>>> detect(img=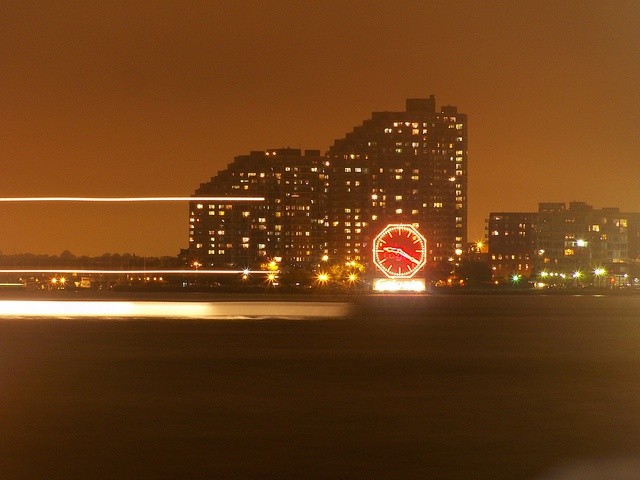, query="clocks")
[373,224,427,280]
